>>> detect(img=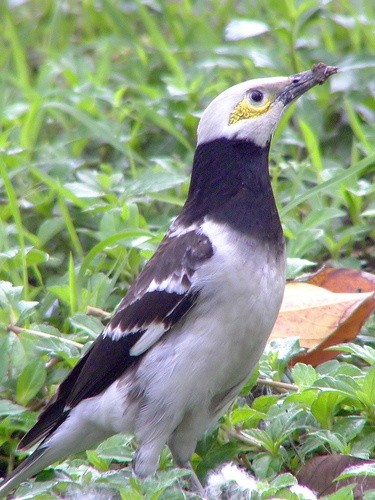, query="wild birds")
[1,61,339,500]
